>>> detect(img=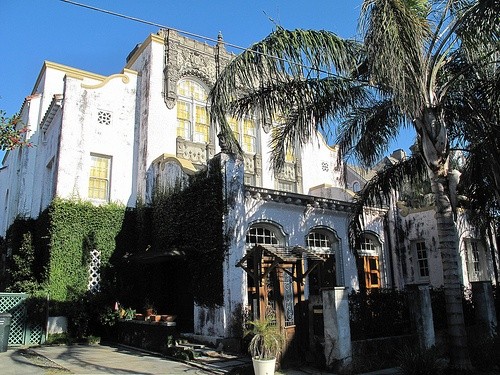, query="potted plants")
[242,315,287,375]
